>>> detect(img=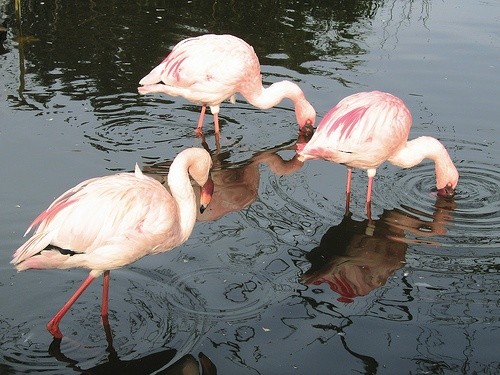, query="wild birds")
[9,147,214,339]
[137,34,316,138]
[128,133,307,222]
[295,90,459,202]
[297,203,458,305]
